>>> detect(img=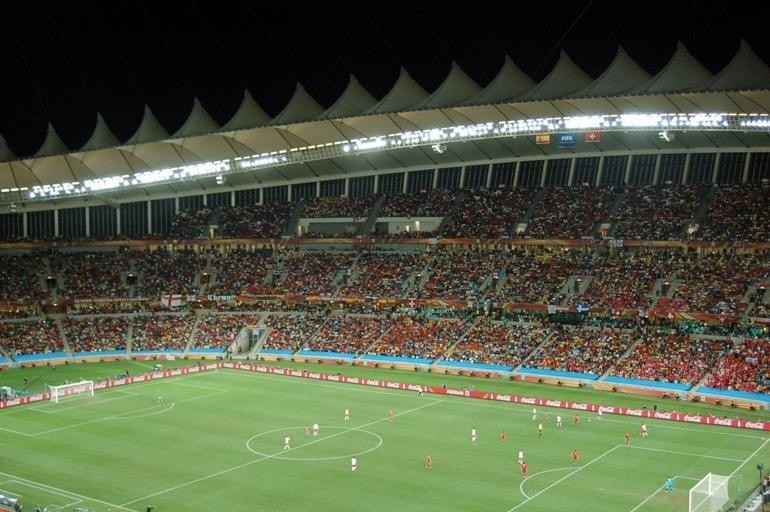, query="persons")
[313,421,319,436]
[521,461,527,479]
[570,449,579,465]
[283,434,291,450]
[574,413,579,424]
[499,430,506,443]
[640,421,644,435]
[624,431,631,448]
[665,475,675,494]
[35,503,41,511]
[537,421,544,435]
[544,409,550,423]
[518,447,524,465]
[641,421,649,439]
[146,503,157,511]
[426,453,433,469]
[597,404,604,421]
[556,413,562,428]
[157,395,163,407]
[418,387,424,400]
[0,181,769,395]
[344,406,349,422]
[14,498,24,512]
[387,406,393,418]
[471,426,476,442]
[305,422,310,436]
[532,406,538,421]
[351,455,358,471]
